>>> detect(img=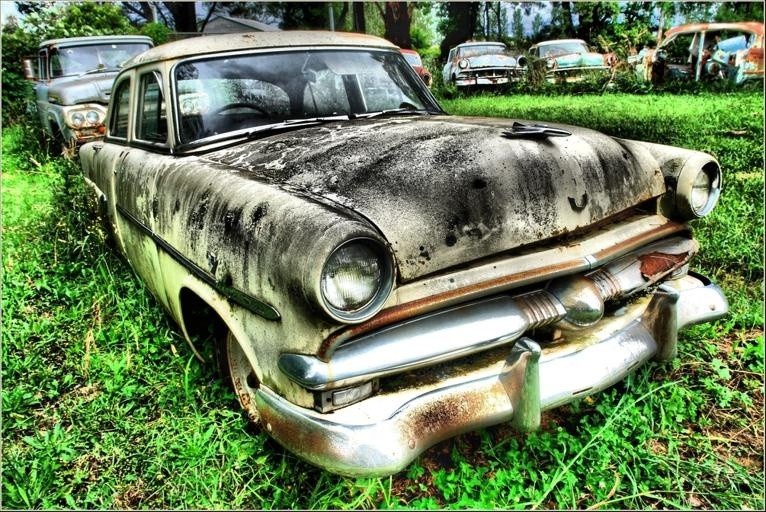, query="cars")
[71,29,733,479]
